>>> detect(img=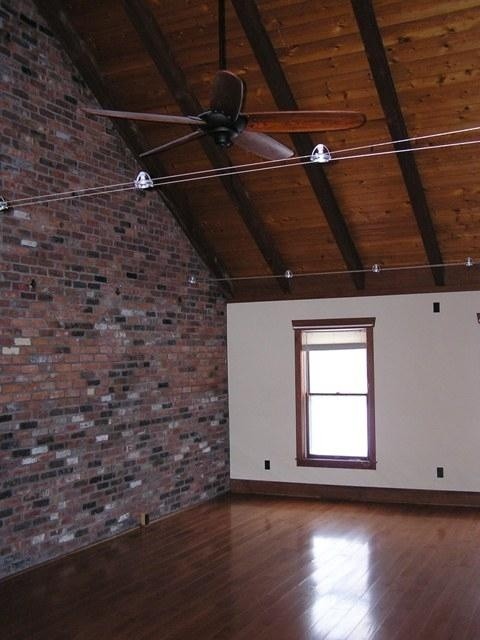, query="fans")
[80,0,366,160]
